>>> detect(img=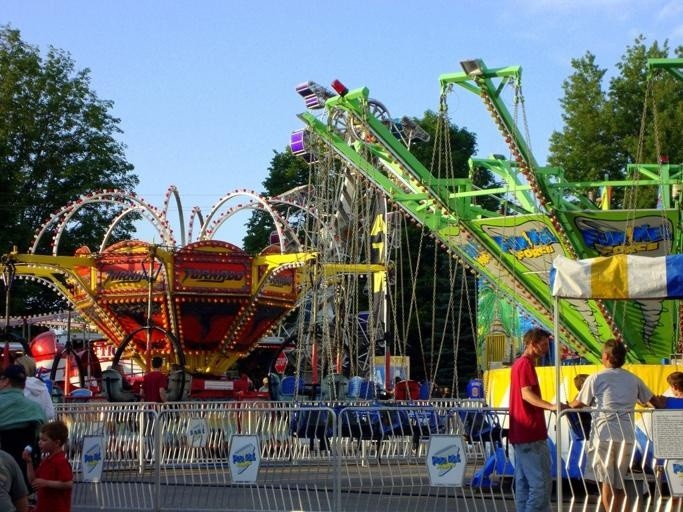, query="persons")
[383,376,401,397]
[662,371,683,409]
[0,355,74,511]
[258,376,269,393]
[507,327,571,512]
[566,338,667,512]
[243,374,254,391]
[574,373,590,400]
[118,354,182,460]
